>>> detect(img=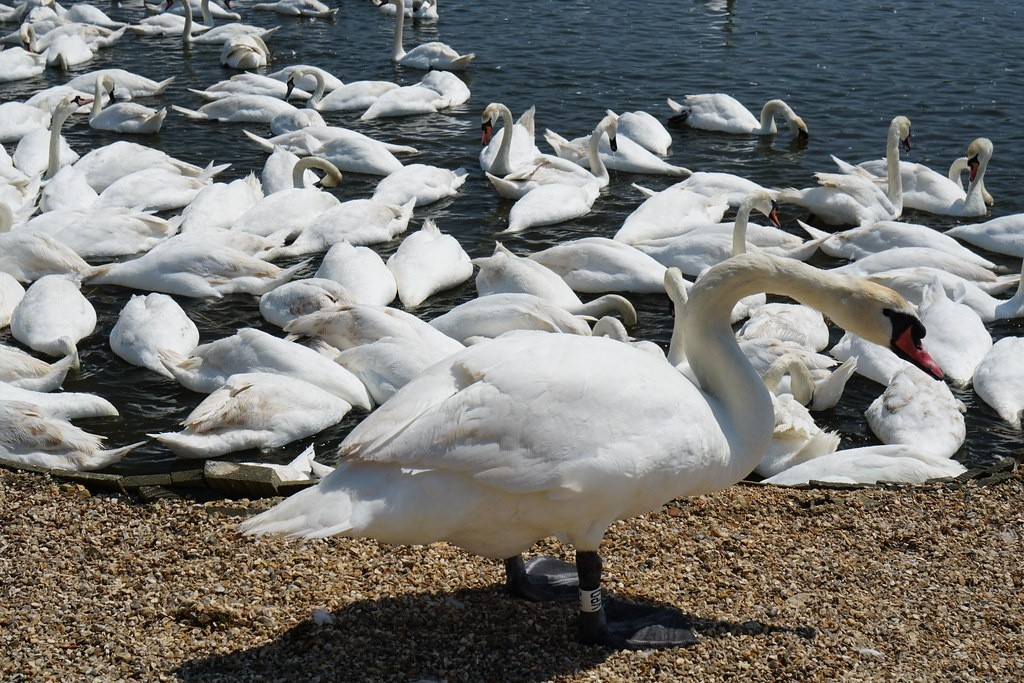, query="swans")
[0,0,1024,649]
